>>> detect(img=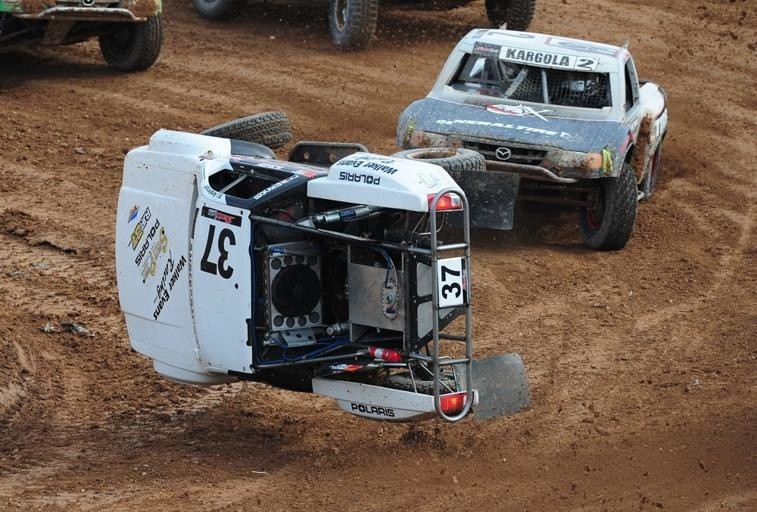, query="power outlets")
[391,22,669,255]
[113,110,534,430]
[189,0,538,55]
[0,0,165,74]
